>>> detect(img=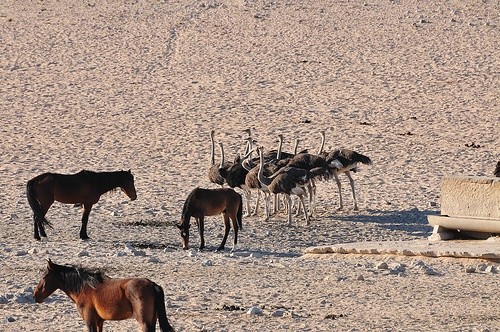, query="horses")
[33,257,176,332]
[26,169,137,240]
[171,185,243,253]
